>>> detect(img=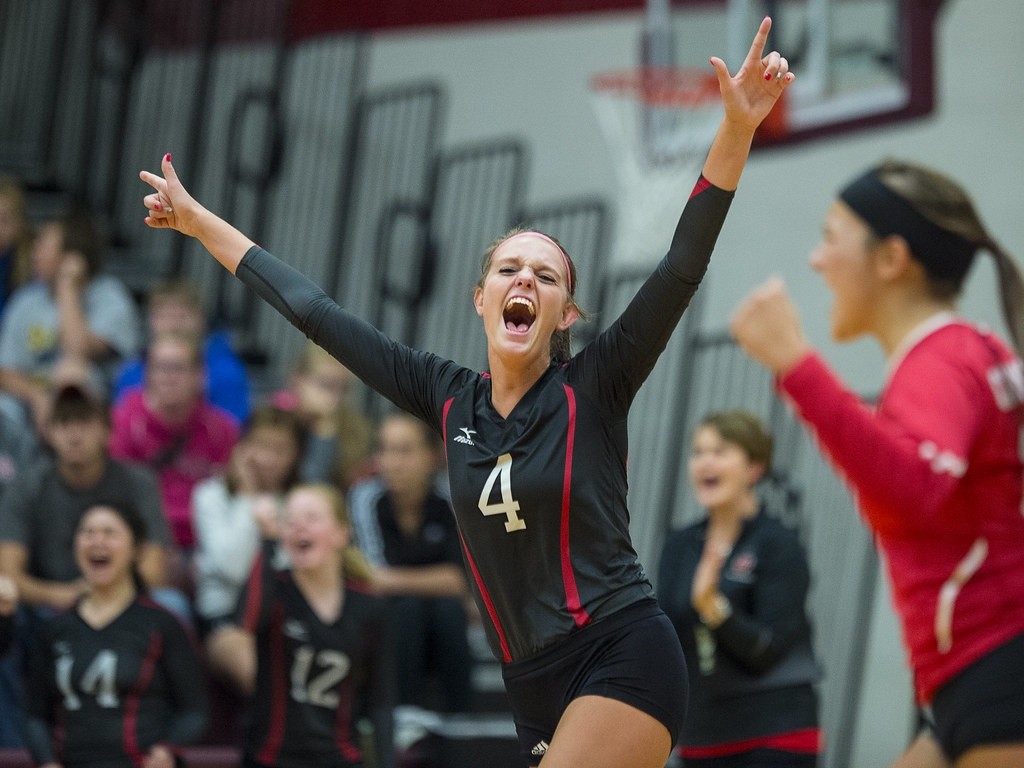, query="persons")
[136,13,799,768]
[653,408,823,768]
[720,159,1024,768]
[0,162,522,768]
